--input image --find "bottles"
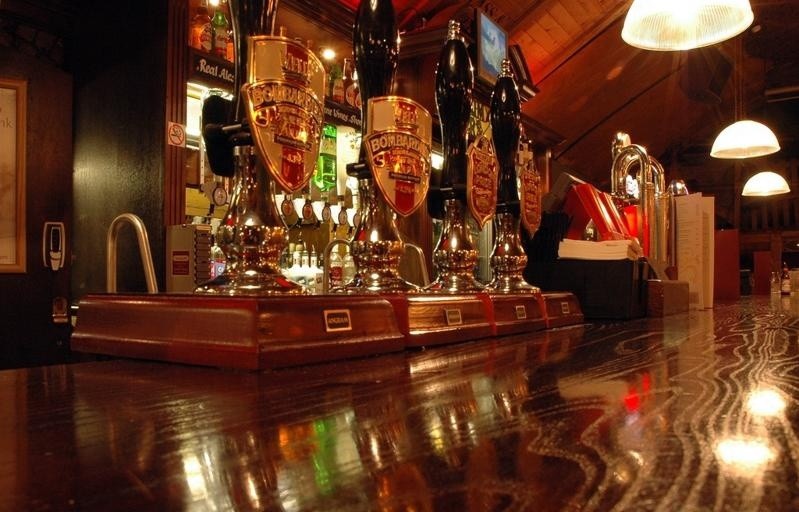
[331,59,345,103]
[330,241,343,287]
[351,66,361,118]
[780,267,791,295]
[210,3,228,59]
[226,30,235,63]
[344,247,357,286]
[279,26,331,99]
[342,57,355,108]
[189,6,213,53]
[769,271,782,295]
[283,238,323,271]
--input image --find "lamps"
[741,171,792,197]
[710,119,781,160]
[621,1,754,54]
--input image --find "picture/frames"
[0,79,29,272]
